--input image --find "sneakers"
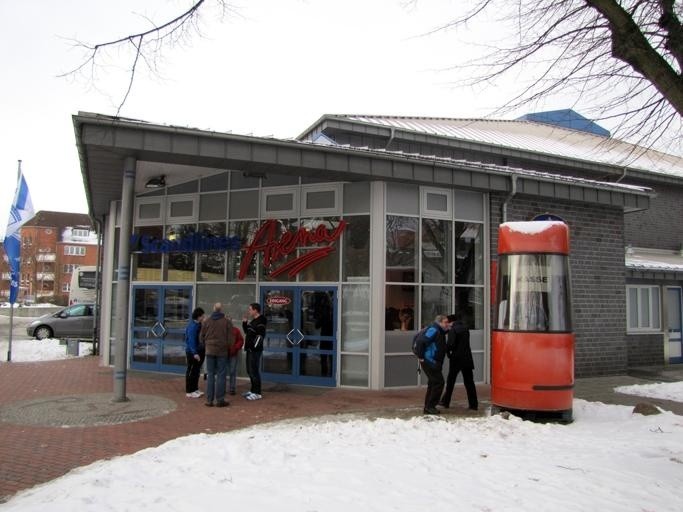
[204,399,229,407]
[438,400,450,409]
[241,390,263,401]
[186,390,205,398]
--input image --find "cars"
[170,252,258,276]
[132,295,320,375]
[24,295,37,305]
[25,301,99,343]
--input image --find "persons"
[438,314,479,411]
[285,291,332,377]
[412,314,449,414]
[199,302,234,407]
[185,307,206,398]
[240,303,267,400]
[225,313,244,395]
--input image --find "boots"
[424,404,441,415]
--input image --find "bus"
[64,265,105,312]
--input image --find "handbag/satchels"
[412,326,432,358]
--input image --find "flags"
[8,178,34,303]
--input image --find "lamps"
[144,172,165,190]
